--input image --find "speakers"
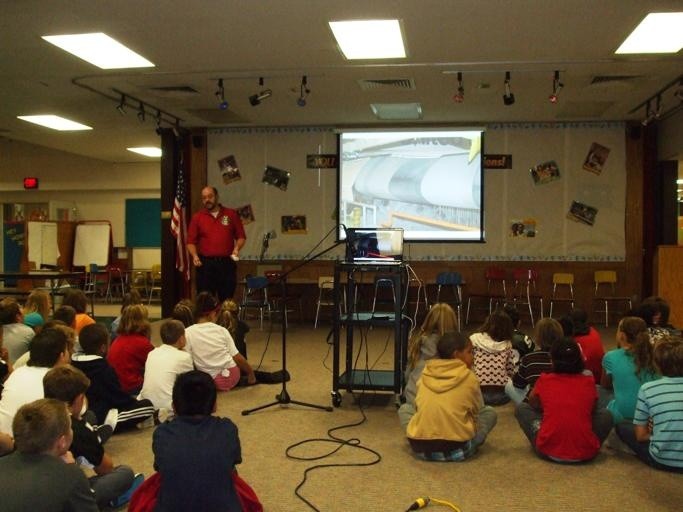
[631,126,641,139]
[193,136,202,148]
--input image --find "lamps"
[440,68,566,105]
[207,69,325,109]
[627,75,683,126]
[113,86,186,137]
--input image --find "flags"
[170,145,191,300]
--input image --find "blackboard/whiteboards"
[125,197,161,249]
[133,247,161,272]
[72,223,111,267]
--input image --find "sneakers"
[75,456,98,479]
[109,473,144,508]
[271,369,290,382]
[136,408,168,429]
[92,424,112,445]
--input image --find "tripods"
[241,241,345,415]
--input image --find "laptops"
[340,227,404,266]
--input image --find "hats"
[23,312,44,327]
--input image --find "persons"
[185,186,246,302]
[509,224,528,237]
[397,297,682,473]
[1,287,291,512]
[531,163,557,182]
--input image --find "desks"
[0,272,84,315]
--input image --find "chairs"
[85,264,165,319]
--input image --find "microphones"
[343,225,358,256]
